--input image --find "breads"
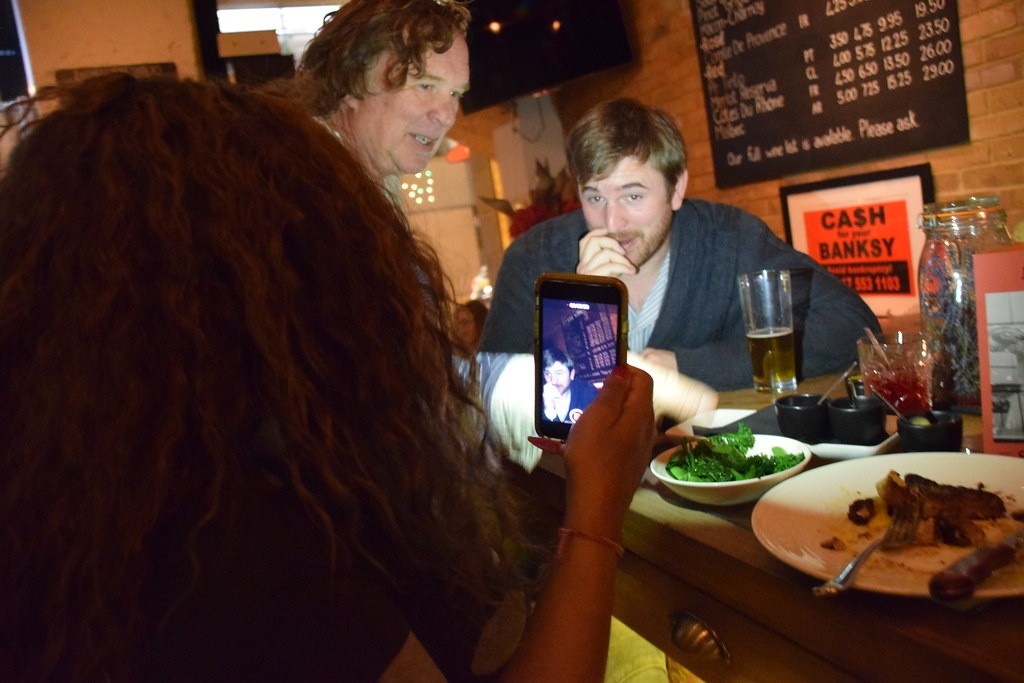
[882,471,1006,547]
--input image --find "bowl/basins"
[650,434,812,506]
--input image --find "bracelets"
[559,527,625,557]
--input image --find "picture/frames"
[780,161,938,317]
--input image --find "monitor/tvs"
[455,0,641,116]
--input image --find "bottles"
[918,194,1011,416]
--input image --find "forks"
[812,497,919,597]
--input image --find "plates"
[751,451,1024,599]
[664,408,899,463]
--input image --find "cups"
[896,411,963,453]
[775,393,832,444]
[827,395,886,445]
[735,268,798,394]
[857,333,932,416]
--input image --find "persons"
[297,0,716,469]
[0,70,662,683]
[540,347,600,424]
[452,300,488,348]
[483,97,887,392]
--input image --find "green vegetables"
[664,421,804,483]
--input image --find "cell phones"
[532,273,630,437]
